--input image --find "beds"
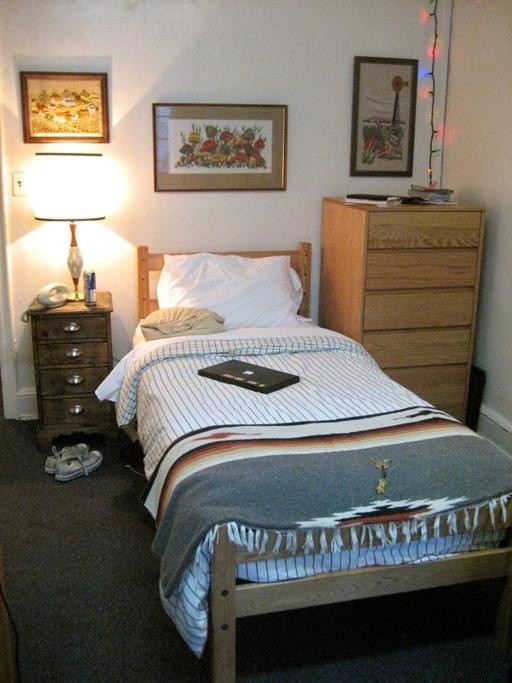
[127,240,509,681]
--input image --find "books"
[344,194,402,207]
[408,189,455,204]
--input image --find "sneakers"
[43,443,103,481]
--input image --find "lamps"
[29,151,110,300]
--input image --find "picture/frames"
[151,102,289,193]
[17,68,111,146]
[350,53,420,179]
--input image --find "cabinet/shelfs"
[320,194,487,428]
[26,289,117,455]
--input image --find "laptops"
[198,358,299,394]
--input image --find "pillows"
[154,250,303,333]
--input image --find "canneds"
[84,272,96,306]
